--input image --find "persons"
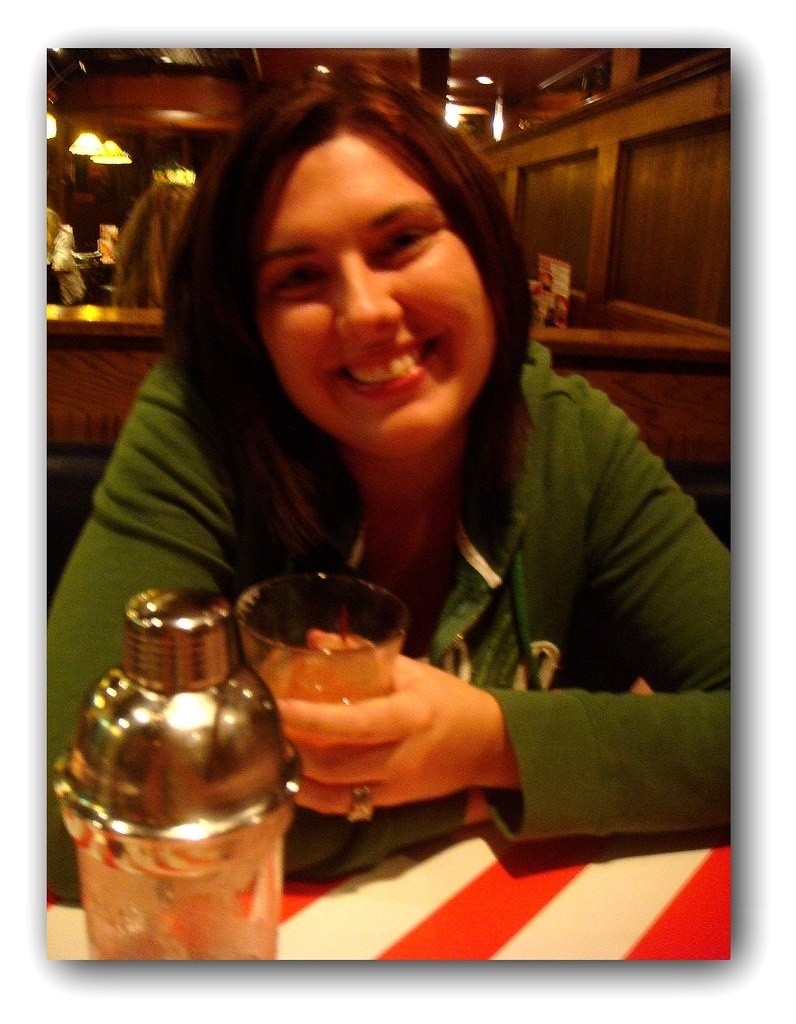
[46,63,730,904]
[112,180,194,307]
[47,206,87,307]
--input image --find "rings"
[347,785,373,822]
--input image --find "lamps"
[67,128,133,164]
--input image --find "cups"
[234,572,407,698]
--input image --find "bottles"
[54,587,301,960]
